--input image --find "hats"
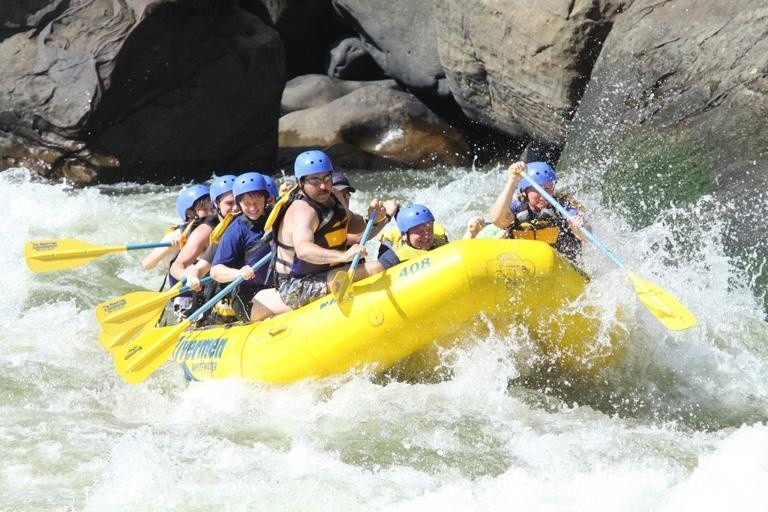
[332,172,355,193]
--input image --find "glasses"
[306,174,332,185]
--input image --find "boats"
[94,238,636,388]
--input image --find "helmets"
[516,162,555,191]
[396,204,435,232]
[176,172,278,220]
[294,149,333,178]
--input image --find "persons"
[490,159,593,269]
[144,171,397,325]
[376,205,487,271]
[272,151,387,312]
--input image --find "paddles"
[26,237,177,273]
[114,249,276,386]
[329,203,382,317]
[94,275,213,336]
[510,165,696,332]
[100,278,190,357]
